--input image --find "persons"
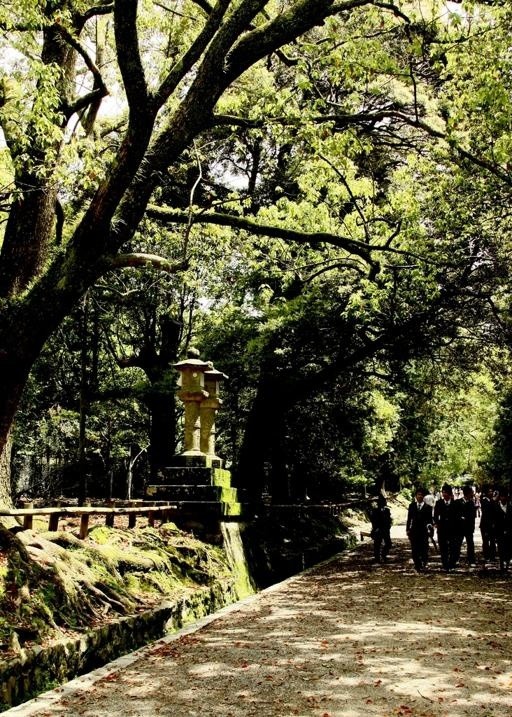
[406,474,512,571]
[369,494,394,563]
